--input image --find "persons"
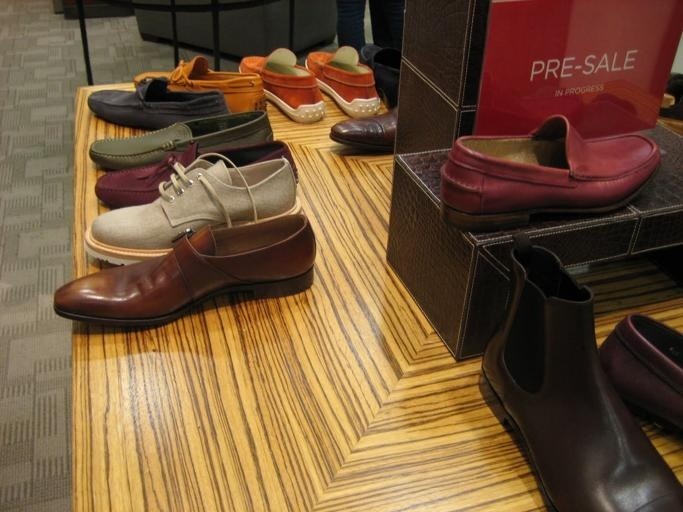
[336,0,404,51]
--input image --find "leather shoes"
[436,113,662,235]
[306,43,382,119]
[331,106,397,150]
[52,212,317,329]
[239,47,324,123]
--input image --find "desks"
[67,56,681,512]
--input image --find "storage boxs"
[378,120,682,370]
[391,1,498,170]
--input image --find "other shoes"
[359,43,402,102]
[81,42,303,267]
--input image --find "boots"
[475,231,683,512]
